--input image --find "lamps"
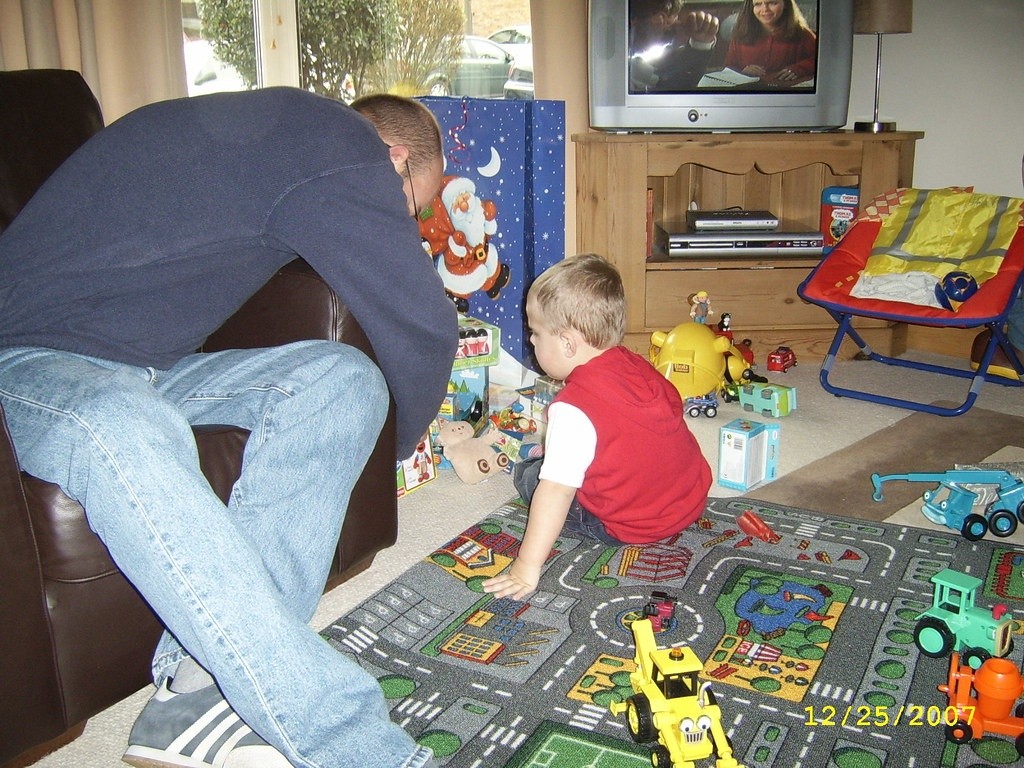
[852,0,913,130]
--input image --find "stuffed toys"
[437,421,509,485]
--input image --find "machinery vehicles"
[869,461,1024,543]
[912,567,1015,671]
[612,616,748,768]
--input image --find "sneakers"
[122,676,295,768]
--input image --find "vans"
[767,345,798,373]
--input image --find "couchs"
[0,69,398,767]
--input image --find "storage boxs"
[396,310,560,498]
[718,419,780,490]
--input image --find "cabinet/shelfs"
[568,129,932,361]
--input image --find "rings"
[789,70,792,72]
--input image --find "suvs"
[721,380,741,403]
[686,392,720,419]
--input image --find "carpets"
[318,490,1024,768]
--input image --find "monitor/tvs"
[588,0,853,133]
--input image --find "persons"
[723,0,816,81]
[690,291,713,324]
[629,0,720,90]
[481,252,713,603]
[0,87,460,768]
